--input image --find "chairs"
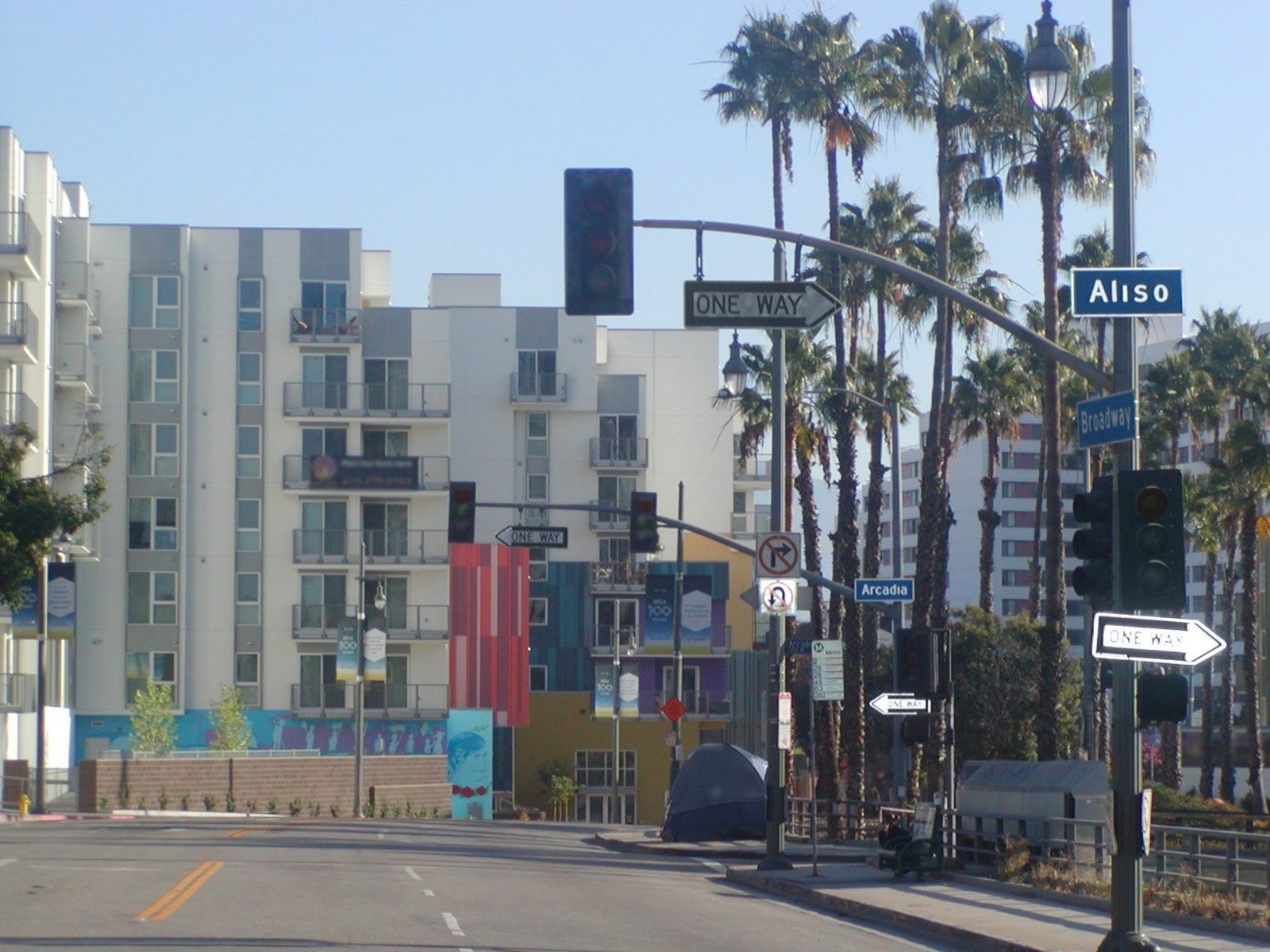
[340,315,357,334]
[292,314,309,333]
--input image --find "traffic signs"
[682,280,844,332]
[868,693,931,717]
[494,525,569,549]
[1090,609,1228,667]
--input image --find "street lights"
[609,593,639,826]
[722,242,793,869]
[1018,0,1161,952]
[350,541,387,819]
[804,386,908,813]
[31,475,76,815]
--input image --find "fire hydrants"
[16,794,32,818]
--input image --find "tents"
[659,741,769,846]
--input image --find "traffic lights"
[629,489,660,555]
[1117,468,1188,611]
[1071,474,1115,610]
[447,480,476,545]
[1135,672,1191,723]
[561,167,635,317]
